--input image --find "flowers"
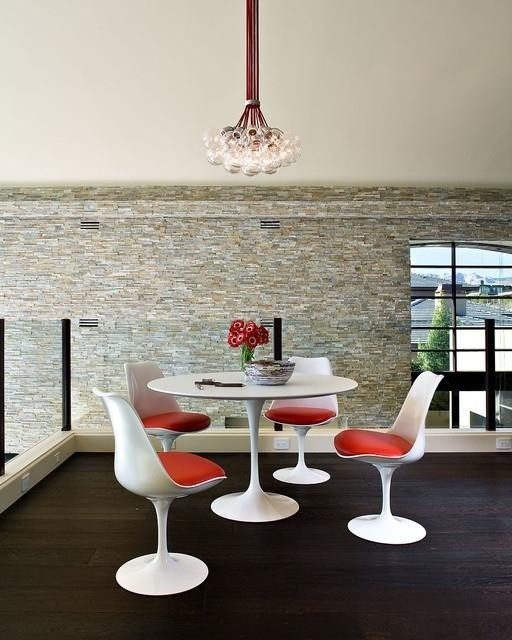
[226,317,270,369]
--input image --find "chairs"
[90,361,228,597]
[264,355,447,546]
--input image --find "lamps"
[200,0,306,182]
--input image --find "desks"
[147,371,359,524]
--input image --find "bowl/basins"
[243,360,296,386]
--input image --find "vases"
[239,344,254,372]
